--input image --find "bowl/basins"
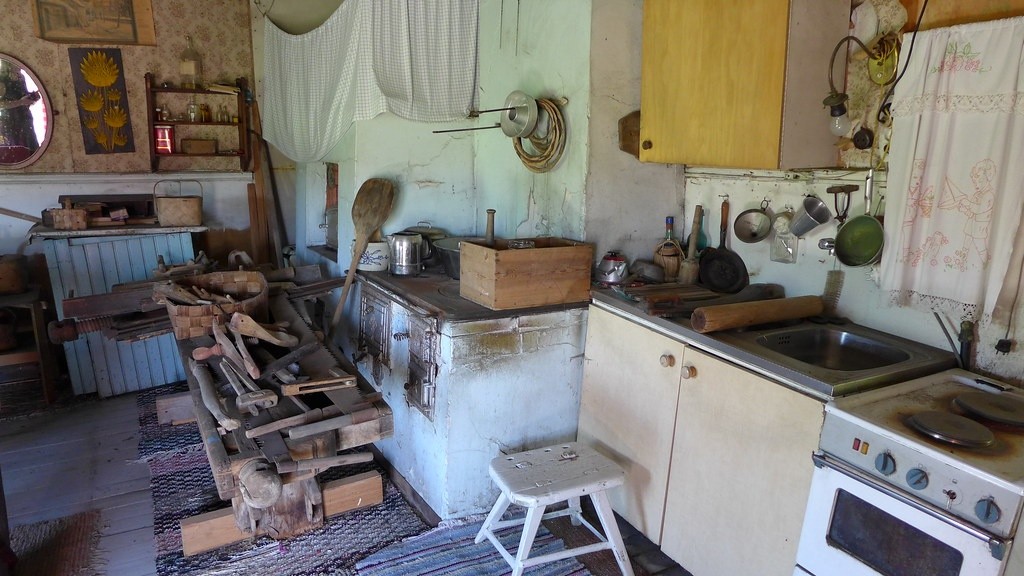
[351,239,390,271]
[403,221,448,267]
[432,236,502,280]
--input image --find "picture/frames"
[36,0,139,44]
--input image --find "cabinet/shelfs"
[145,71,251,172]
[638,0,852,170]
[0,296,59,418]
[575,304,832,576]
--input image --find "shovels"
[326,177,394,341]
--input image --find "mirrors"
[0,52,53,170]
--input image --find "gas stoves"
[818,367,1024,539]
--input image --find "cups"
[787,197,830,237]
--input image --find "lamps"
[823,34,898,136]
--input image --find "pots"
[834,168,885,267]
[699,200,750,294]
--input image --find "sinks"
[752,323,913,371]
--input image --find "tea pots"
[594,251,629,285]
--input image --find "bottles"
[661,216,675,246]
[179,35,203,93]
[161,95,238,123]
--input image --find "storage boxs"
[460,237,593,310]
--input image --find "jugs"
[385,232,422,277]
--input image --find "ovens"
[794,450,1012,576]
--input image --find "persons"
[0,60,40,151]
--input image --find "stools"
[475,442,635,576]
